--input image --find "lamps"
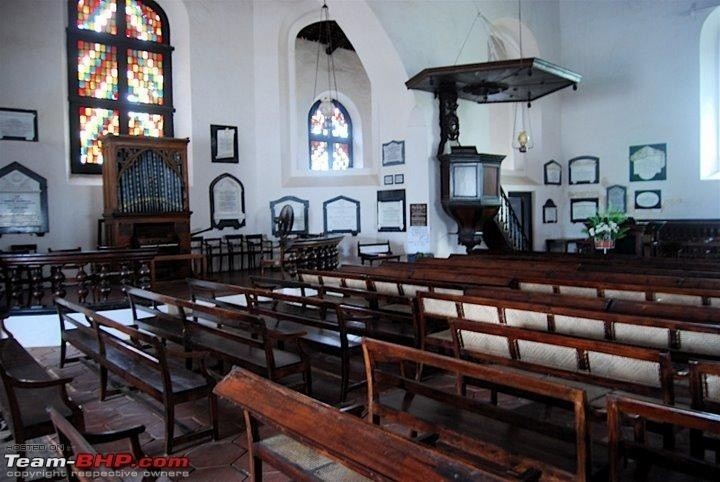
[314,1,346,124]
[509,1,537,154]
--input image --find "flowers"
[579,201,633,242]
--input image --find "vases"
[592,234,617,254]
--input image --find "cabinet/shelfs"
[435,148,508,212]
